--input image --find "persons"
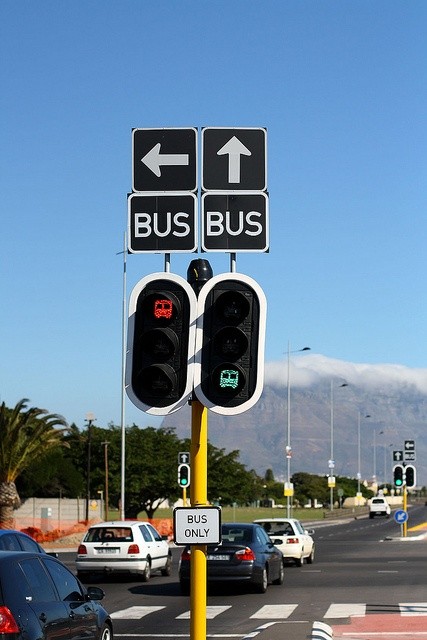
[263,523,272,533]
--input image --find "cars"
[0,531,58,559]
[75,521,172,581]
[0,552,113,640]
[368,498,390,518]
[178,523,284,591]
[253,518,315,566]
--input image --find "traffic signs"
[127,127,198,253]
[404,440,415,462]
[178,452,189,464]
[172,507,220,545]
[395,510,408,524]
[392,451,402,461]
[200,126,269,253]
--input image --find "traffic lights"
[124,272,196,415]
[392,465,404,488]
[404,465,416,488]
[177,464,190,488]
[193,273,266,415]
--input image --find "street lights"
[329,379,347,510]
[357,411,370,505]
[384,443,393,495]
[83,413,97,520]
[283,339,310,519]
[373,429,385,497]
[101,441,112,521]
